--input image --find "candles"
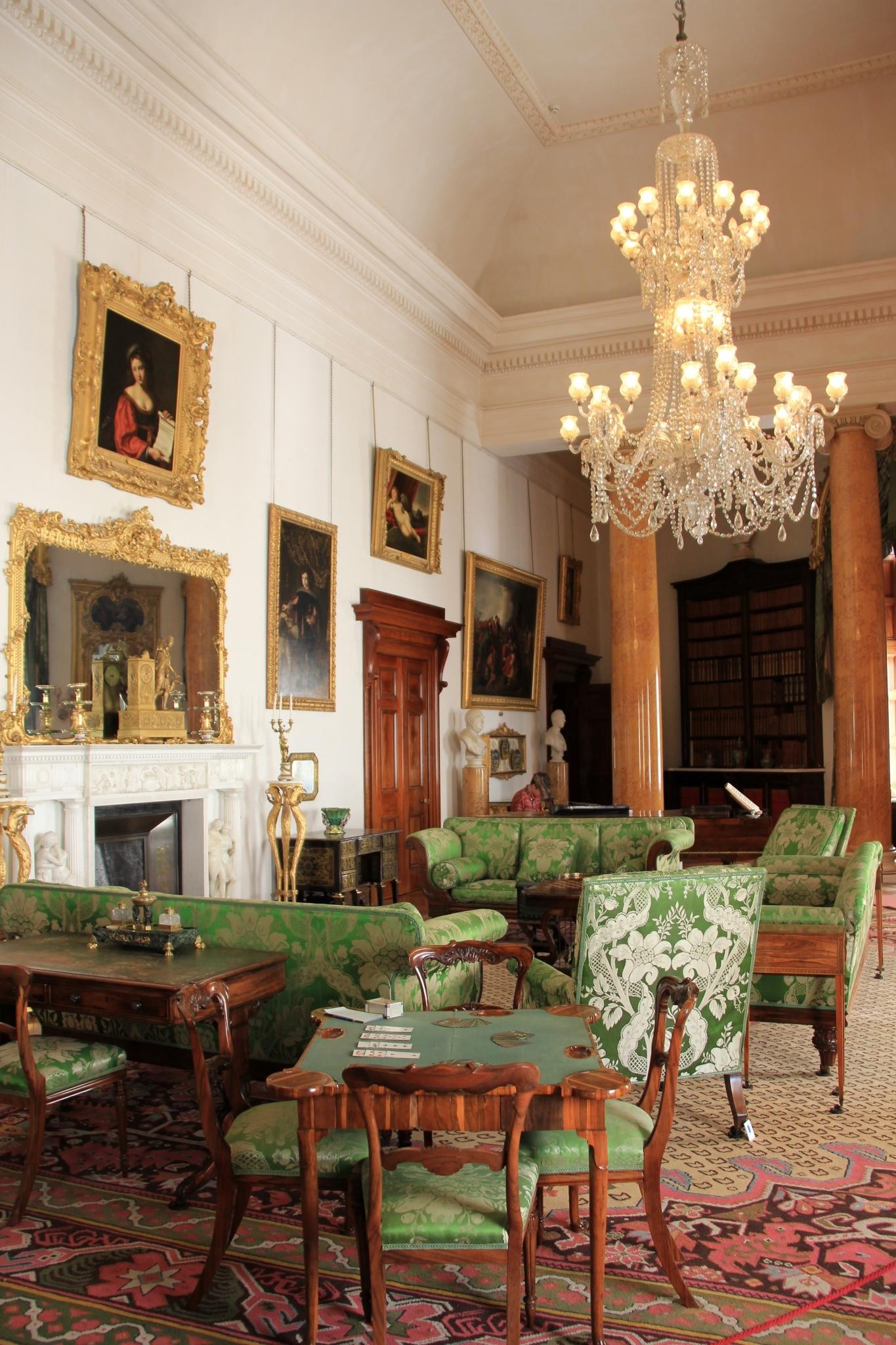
[273,692,293,720]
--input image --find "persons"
[459,709,488,767]
[510,772,552,812]
[151,636,181,710]
[543,709,569,762]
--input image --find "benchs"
[406,815,695,919]
[742,841,885,1114]
[0,879,508,1080]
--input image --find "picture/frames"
[266,504,338,712]
[461,551,548,711]
[370,447,447,575]
[557,555,582,626]
[66,260,216,508]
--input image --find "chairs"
[173,865,768,1345]
[762,804,857,857]
[0,964,128,1224]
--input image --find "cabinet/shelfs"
[670,556,823,769]
[275,828,402,906]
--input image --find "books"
[685,585,810,768]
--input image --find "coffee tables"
[515,872,596,967]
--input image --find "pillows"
[616,857,645,873]
[432,857,488,889]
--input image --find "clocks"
[117,651,187,744]
[84,651,124,739]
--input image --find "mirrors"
[0,506,235,745]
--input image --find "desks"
[0,930,289,1210]
[665,768,824,816]
[266,1004,633,1345]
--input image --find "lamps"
[560,0,848,550]
